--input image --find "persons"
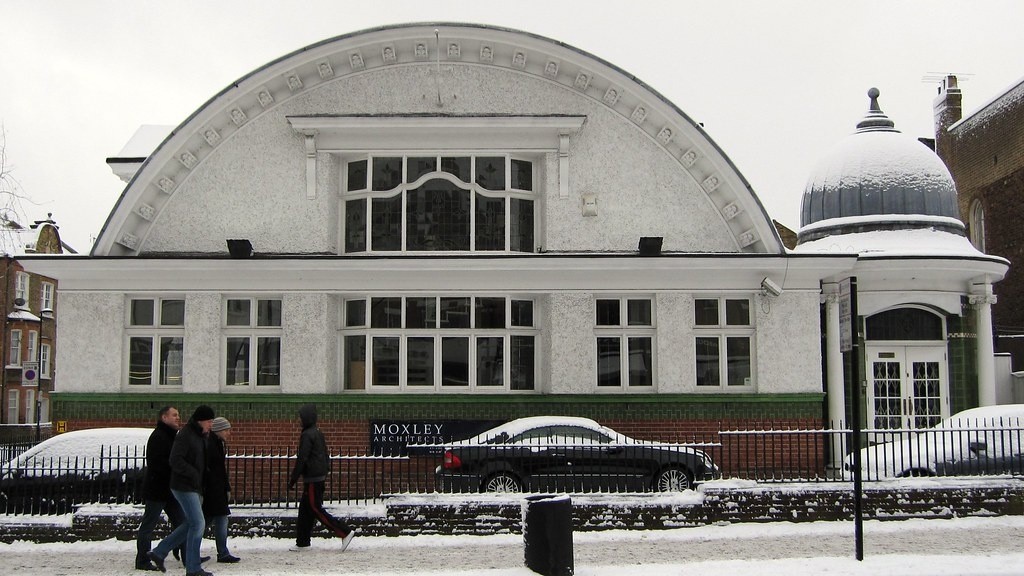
[135,405,211,571]
[173,417,241,564]
[287,406,355,553]
[147,406,215,576]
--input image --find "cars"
[435,416,720,495]
[843,403,1024,480]
[0,428,181,514]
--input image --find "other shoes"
[200,556,210,563]
[341,530,354,552]
[217,555,241,563]
[135,562,158,571]
[186,568,213,576]
[289,544,312,552]
[148,550,167,574]
[172,547,181,561]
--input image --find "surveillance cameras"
[760,276,783,296]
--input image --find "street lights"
[37,308,54,442]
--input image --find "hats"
[211,417,231,432]
[194,405,215,422]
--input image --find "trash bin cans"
[521,493,574,576]
